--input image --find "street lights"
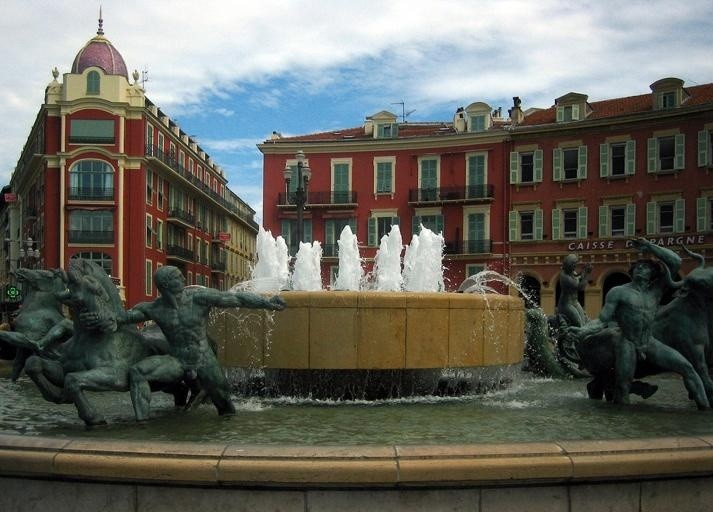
[19,237,40,295]
[283,150,312,250]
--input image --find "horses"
[24,256,218,426]
[0,267,75,385]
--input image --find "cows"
[557,242,713,400]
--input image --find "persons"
[555,254,595,329]
[565,237,710,412]
[125,266,286,422]
